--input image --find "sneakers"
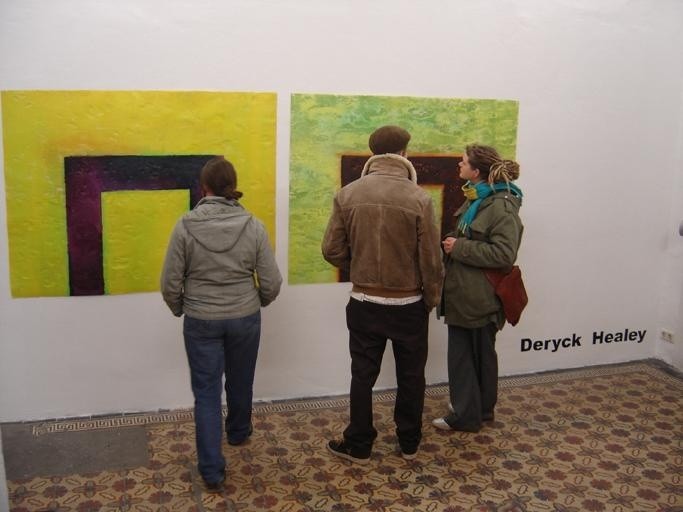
[396,429,420,460]
[327,439,371,466]
[206,467,227,494]
[432,403,455,430]
[228,421,253,445]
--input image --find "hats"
[368,125,411,154]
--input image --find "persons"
[318,125,449,466]
[159,154,282,489]
[432,140,524,433]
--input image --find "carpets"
[2,412,150,479]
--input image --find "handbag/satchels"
[483,265,529,327]
